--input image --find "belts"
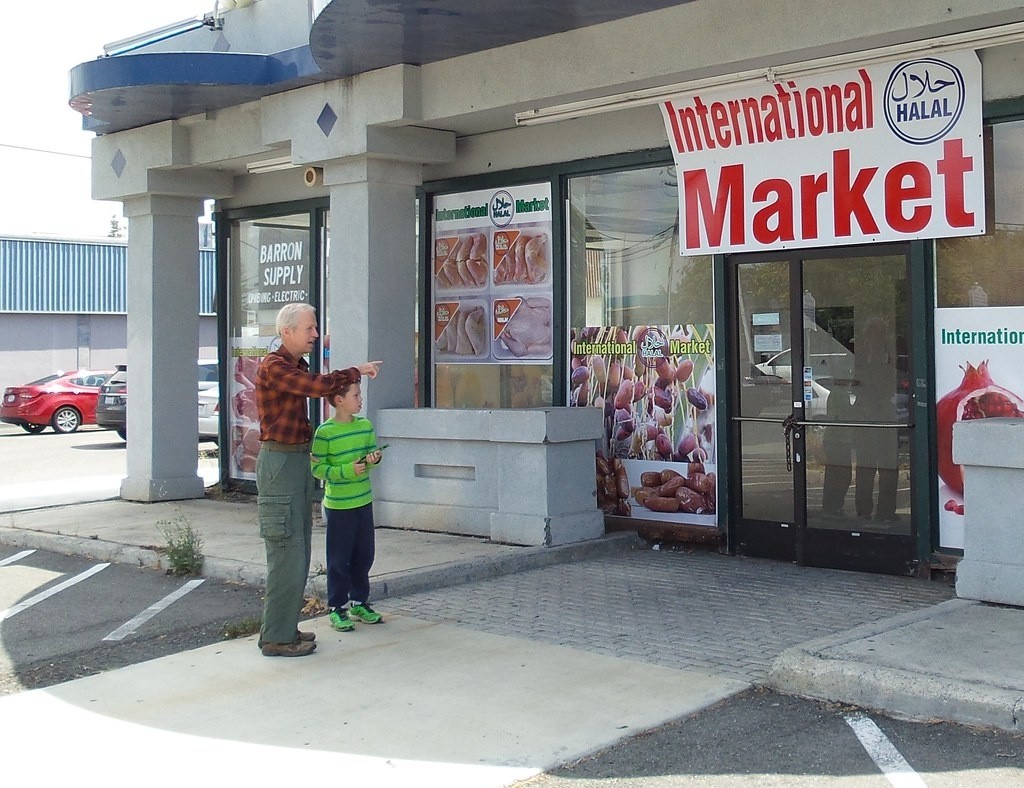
[260,442,311,454]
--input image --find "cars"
[95,364,127,440]
[0,368,113,435]
[752,365,831,441]
[197,358,219,447]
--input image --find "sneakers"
[876,513,900,522]
[329,607,355,632]
[855,513,871,521]
[823,508,847,520]
[349,602,382,624]
[262,641,316,657]
[258,630,315,648]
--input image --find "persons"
[254,303,384,657]
[821,318,901,522]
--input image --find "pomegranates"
[935,358,1024,515]
